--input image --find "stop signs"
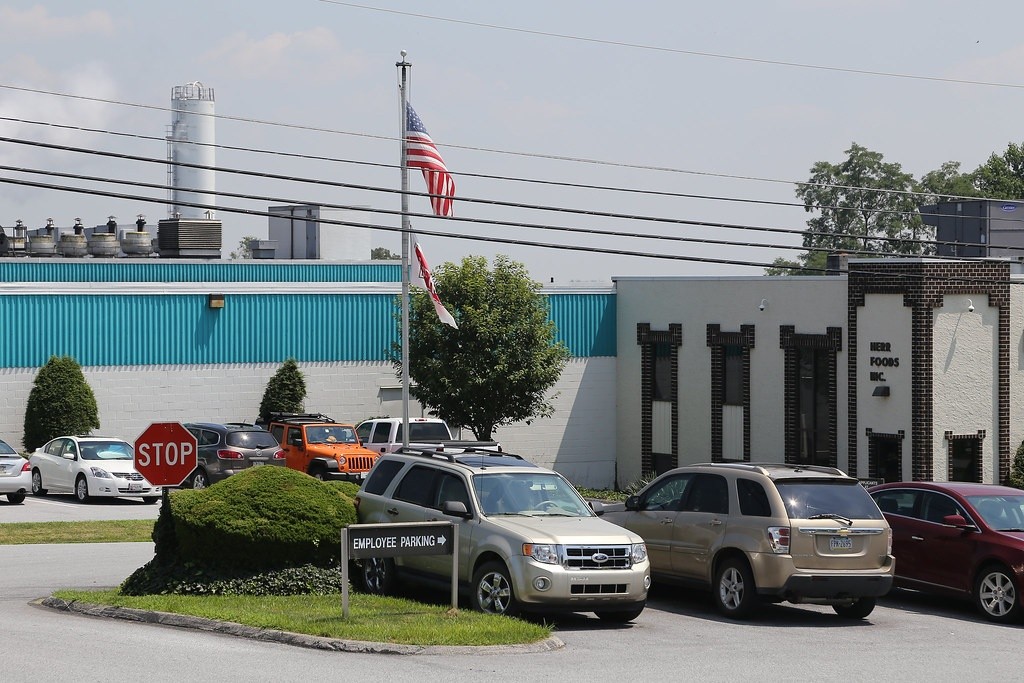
[133,421,198,486]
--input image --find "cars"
[0,437,31,505]
[866,480,1024,630]
[29,435,163,504]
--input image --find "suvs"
[352,441,652,629]
[180,422,287,490]
[266,411,381,481]
[596,459,896,620]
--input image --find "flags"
[410,226,459,329]
[406,100,456,217]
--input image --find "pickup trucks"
[347,417,502,460]
